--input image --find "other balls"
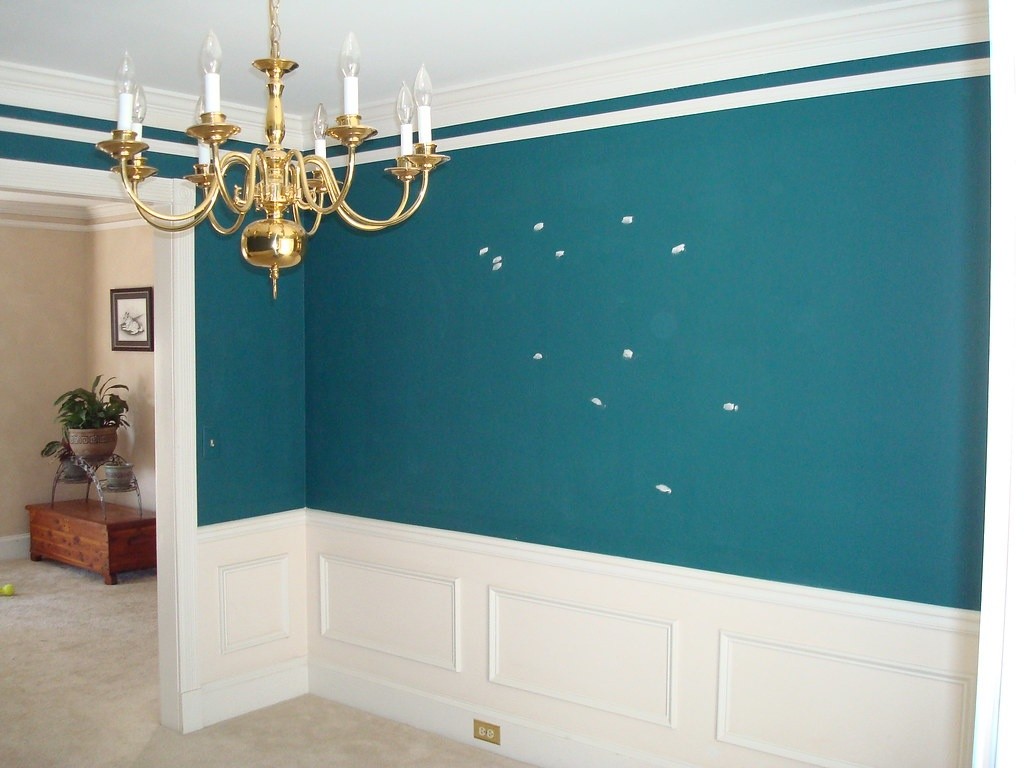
[4,584,14,595]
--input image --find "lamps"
[96,0,451,299]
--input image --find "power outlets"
[474,719,500,745]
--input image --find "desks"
[25,499,156,585]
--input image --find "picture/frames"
[109,287,154,352]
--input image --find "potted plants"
[104,456,135,490]
[54,374,130,461]
[41,440,89,481]
